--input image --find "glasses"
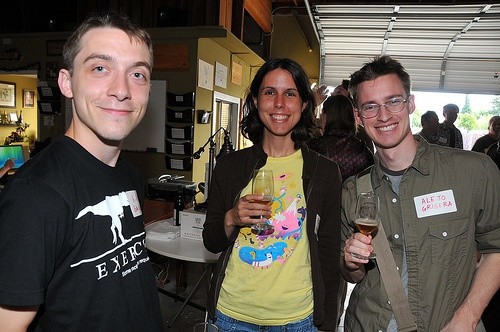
[358,96,409,119]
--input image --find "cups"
[193,323,219,332]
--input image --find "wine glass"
[251,170,274,230]
[351,193,380,259]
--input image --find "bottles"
[173,185,185,226]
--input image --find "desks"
[143,217,222,332]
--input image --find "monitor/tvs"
[0,145,25,170]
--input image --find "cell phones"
[342,79,349,91]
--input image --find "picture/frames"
[0,80,56,134]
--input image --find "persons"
[302,76,375,163]
[471,116,500,165]
[413,110,440,145]
[306,96,376,330]
[202,57,344,332]
[1,11,163,332]
[437,105,462,148]
[338,57,500,332]
